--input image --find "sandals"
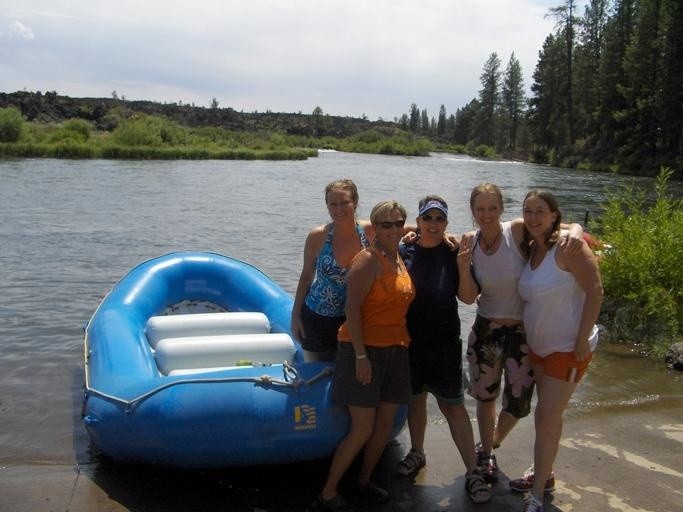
[394,450,427,477]
[479,450,497,482]
[318,495,357,511]
[358,482,390,504]
[474,438,500,453]
[465,469,490,502]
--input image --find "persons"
[511,190,604,512]
[388,196,478,479]
[293,178,423,360]
[463,184,583,507]
[314,202,420,511]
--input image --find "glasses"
[378,220,405,227]
[421,215,446,222]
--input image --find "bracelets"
[355,352,367,359]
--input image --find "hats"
[418,200,449,215]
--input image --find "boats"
[85,250,410,469]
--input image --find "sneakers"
[509,472,554,492]
[522,498,543,512]
